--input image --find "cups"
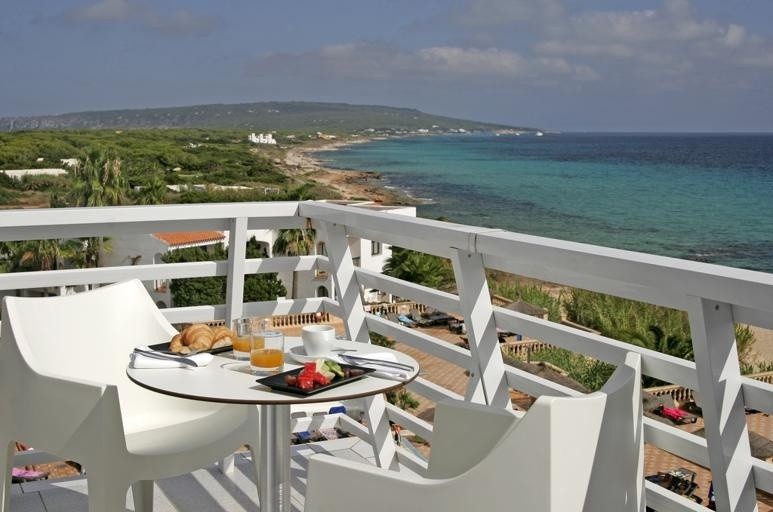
[233,317,267,361]
[302,325,335,356]
[250,331,285,377]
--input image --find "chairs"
[305,351,647,512]
[0,279,262,512]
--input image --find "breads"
[168,322,234,355]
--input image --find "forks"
[337,352,414,371]
[133,348,198,370]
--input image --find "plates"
[288,345,347,364]
[255,364,376,395]
[148,340,233,358]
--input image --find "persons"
[10,444,41,479]
[656,473,672,488]
[657,402,689,422]
